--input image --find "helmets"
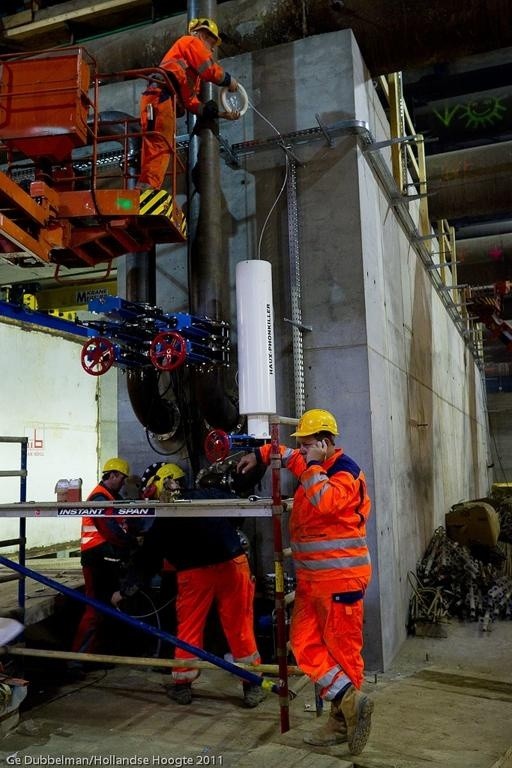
[103,458,130,476]
[147,472,173,498]
[156,464,186,479]
[289,409,338,437]
[188,18,222,47]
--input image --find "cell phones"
[322,439,329,448]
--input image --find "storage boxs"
[445,502,500,548]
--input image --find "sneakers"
[302,699,347,747]
[175,684,191,704]
[339,684,374,753]
[244,682,266,706]
[134,182,156,190]
[67,659,87,680]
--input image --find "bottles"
[55,477,83,502]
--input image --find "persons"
[139,461,279,704]
[138,15,242,193]
[67,454,131,670]
[235,404,376,757]
